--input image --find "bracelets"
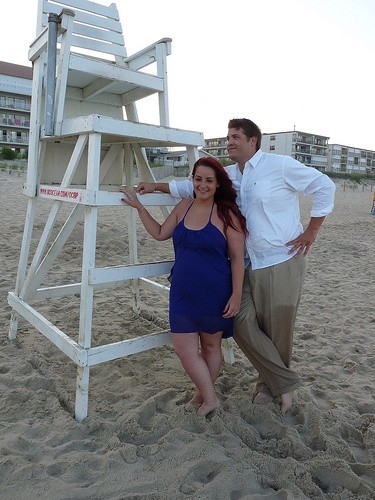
[138,206,144,213]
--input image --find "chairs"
[27,0,173,139]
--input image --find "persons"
[117,156,250,418]
[135,118,336,416]
